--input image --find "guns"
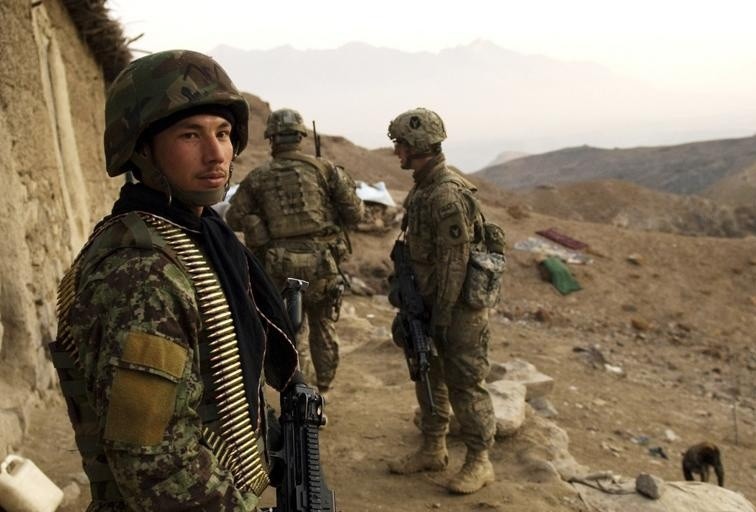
[311,122,323,158]
[270,276,336,508]
[391,239,439,419]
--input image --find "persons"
[45,49,296,511]
[386,106,496,493]
[223,110,359,428]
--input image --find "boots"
[448,449,495,493]
[388,434,449,476]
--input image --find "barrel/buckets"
[0,454,63,511]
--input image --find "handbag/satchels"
[467,251,505,310]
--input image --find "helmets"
[387,107,446,156]
[103,49,249,177]
[264,108,307,142]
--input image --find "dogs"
[679,440,724,487]
